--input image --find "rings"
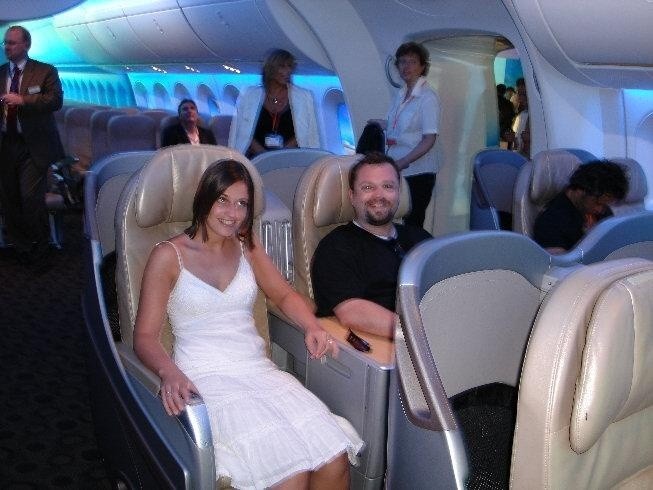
[164,391,171,395]
[326,339,332,344]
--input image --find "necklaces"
[266,87,285,104]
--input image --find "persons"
[533,159,629,255]
[366,42,440,229]
[224,48,320,162]
[496,77,530,160]
[308,151,439,340]
[160,98,219,148]
[132,157,368,490]
[0,25,66,267]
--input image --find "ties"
[5,67,20,147]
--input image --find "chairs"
[56,103,653,489]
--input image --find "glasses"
[1,39,24,47]
